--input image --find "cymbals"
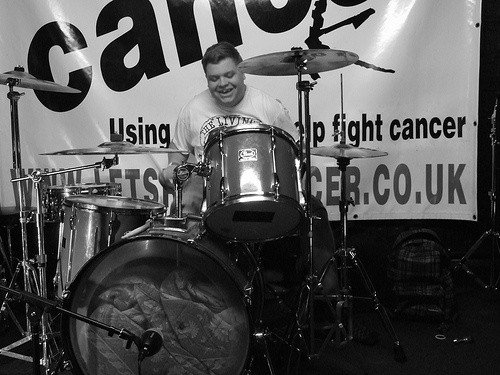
[310,143,388,159]
[1,71,83,94]
[38,139,189,156]
[236,50,360,76]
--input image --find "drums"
[55,225,266,375]
[54,194,166,302]
[201,121,305,246]
[46,184,122,222]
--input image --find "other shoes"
[341,318,378,345]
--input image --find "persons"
[167,41,379,345]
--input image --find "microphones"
[138,328,164,361]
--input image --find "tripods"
[0,78,120,375]
[453,100,500,295]
[279,56,406,375]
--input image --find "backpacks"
[386,219,456,323]
